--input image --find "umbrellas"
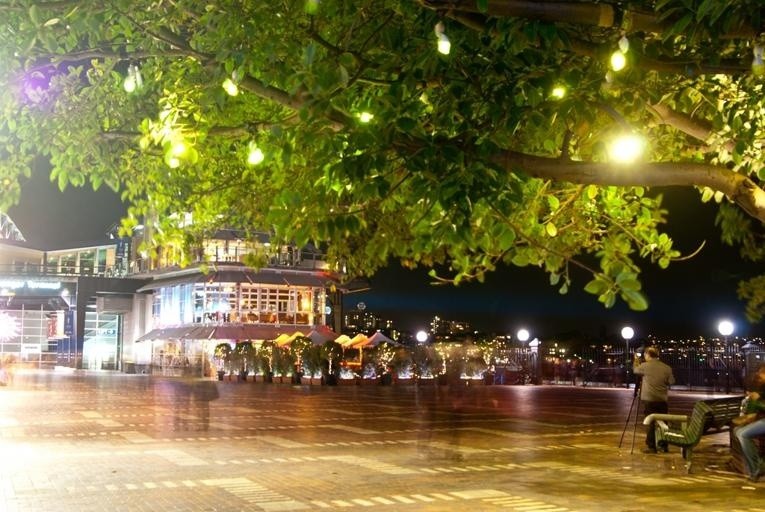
[270,327,399,364]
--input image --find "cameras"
[636,352,644,357]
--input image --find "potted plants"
[214,336,507,386]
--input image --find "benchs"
[655,396,760,475]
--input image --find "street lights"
[621,326,634,388]
[718,321,734,394]
[517,329,530,384]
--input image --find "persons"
[733,362,765,483]
[633,346,676,454]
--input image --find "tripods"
[618,361,643,455]
